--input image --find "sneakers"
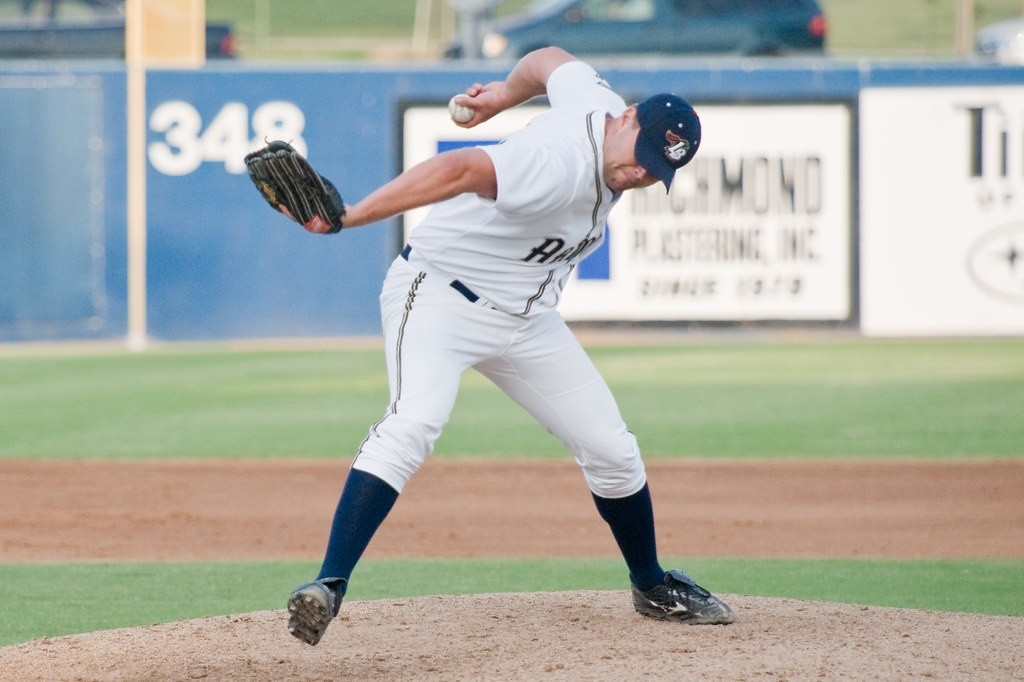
[632,567,736,624]
[287,577,347,646]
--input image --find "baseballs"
[448,93,474,122]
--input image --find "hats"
[634,94,701,194]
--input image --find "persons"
[244,46,737,647]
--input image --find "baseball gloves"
[244,140,348,234]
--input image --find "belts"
[400,244,480,303]
